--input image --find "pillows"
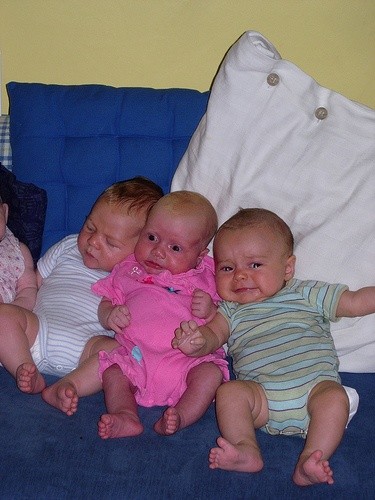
[7,81,212,260]
[170,31,375,373]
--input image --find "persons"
[171,208,375,485]
[0,190,37,312]
[92,190,229,439]
[0,176,165,415]
